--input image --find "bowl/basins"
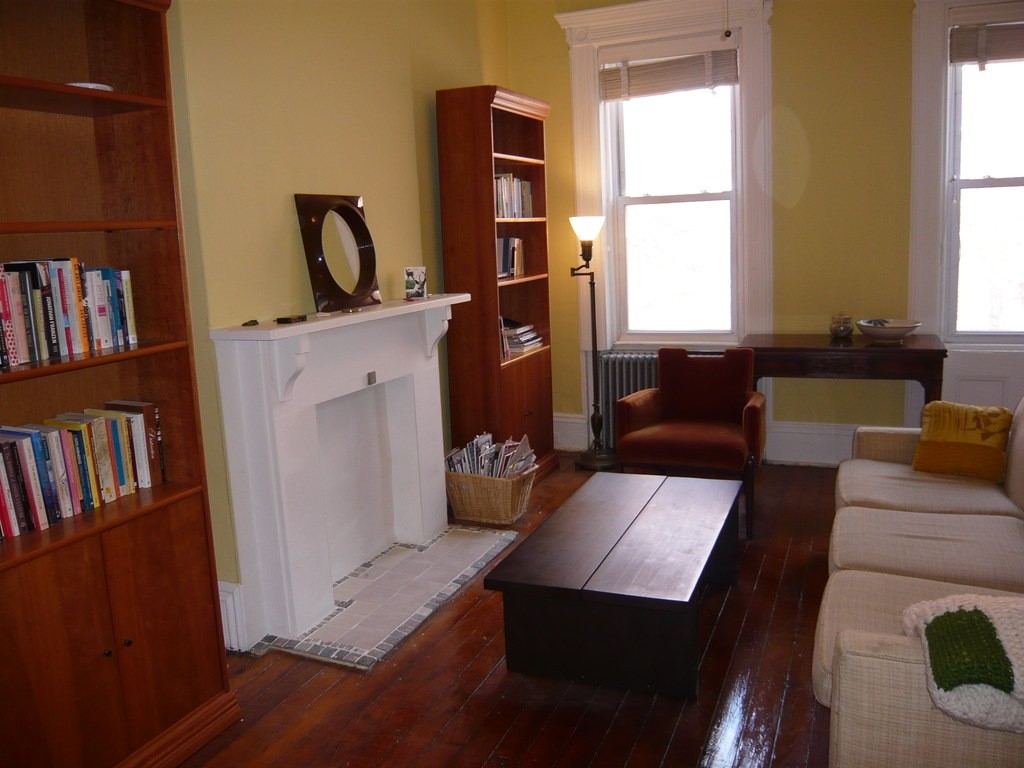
[856,319,922,342]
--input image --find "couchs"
[811,394,1024,768]
[615,347,766,540]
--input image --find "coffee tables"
[483,471,743,704]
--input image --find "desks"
[738,333,948,426]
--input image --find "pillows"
[900,592,1024,734]
[912,400,1013,482]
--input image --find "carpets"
[246,524,517,670]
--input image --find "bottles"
[830,315,854,338]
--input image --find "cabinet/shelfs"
[436,84,561,487]
[0,0,228,768]
[208,290,472,638]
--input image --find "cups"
[404,266,427,301]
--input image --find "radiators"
[598,351,659,450]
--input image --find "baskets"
[446,463,539,525]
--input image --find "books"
[443,433,537,478]
[498,238,524,278]
[0,399,167,540]
[0,256,138,368]
[494,173,532,218]
[504,324,544,352]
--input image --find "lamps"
[569,215,616,471]
